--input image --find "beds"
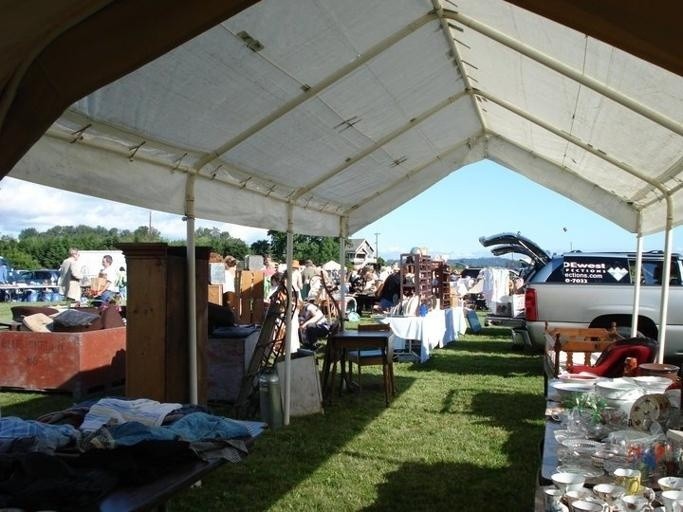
[543,320,629,398]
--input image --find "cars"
[479,230,683,358]
[461,266,519,279]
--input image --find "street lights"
[560,224,573,251]
[512,230,522,260]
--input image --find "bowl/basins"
[550,363,680,406]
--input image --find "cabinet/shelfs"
[430,261,451,310]
[400,253,433,312]
[112,241,212,408]
[1,324,127,391]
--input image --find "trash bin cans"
[42,292,59,301]
[26,290,37,302]
[15,292,23,301]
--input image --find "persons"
[115,266,127,300]
[207,247,464,352]
[58,247,83,308]
[510,267,529,295]
[88,255,119,310]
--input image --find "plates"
[553,388,683,479]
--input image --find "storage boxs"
[510,293,526,318]
[491,299,510,316]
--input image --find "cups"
[546,467,683,512]
[420,303,427,317]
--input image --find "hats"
[292,260,302,269]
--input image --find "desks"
[442,306,467,345]
[545,377,571,422]
[538,422,667,491]
[536,482,592,511]
[328,330,396,407]
[369,309,446,363]
[0,283,61,302]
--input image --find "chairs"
[566,337,658,377]
[348,322,396,396]
